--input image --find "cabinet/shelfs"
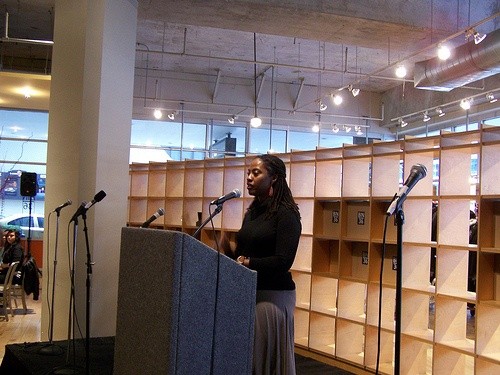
[127,124,500,375]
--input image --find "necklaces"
[1,246,12,257]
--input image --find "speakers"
[20,172,38,196]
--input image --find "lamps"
[228,115,238,124]
[354,125,364,137]
[423,110,431,121]
[438,108,445,117]
[343,124,351,133]
[330,93,342,105]
[489,92,498,104]
[332,124,339,133]
[167,110,179,121]
[400,118,408,127]
[317,100,328,113]
[469,28,488,45]
[347,85,361,98]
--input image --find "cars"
[0,212,44,240]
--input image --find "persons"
[220,154,302,375]
[0,229,24,308]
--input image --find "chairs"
[11,257,40,312]
[0,261,20,322]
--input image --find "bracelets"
[8,263,10,267]
[237,256,243,264]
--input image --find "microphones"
[78,191,106,215]
[70,200,86,221]
[54,200,72,211]
[386,164,428,215]
[210,189,242,204]
[141,207,166,228]
